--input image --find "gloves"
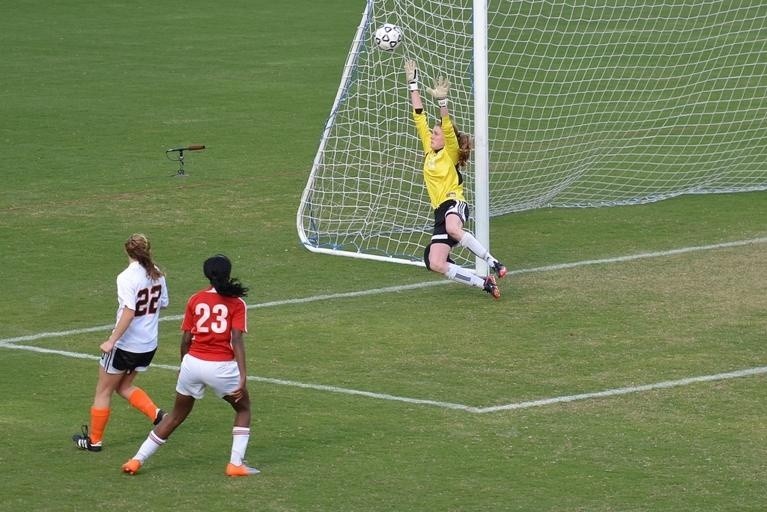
[426,75,450,107]
[403,60,419,91]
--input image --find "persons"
[403,58,507,300]
[120,253,260,476]
[71,232,170,451]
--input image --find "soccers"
[373,23,403,50]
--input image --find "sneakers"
[491,261,509,280]
[481,274,500,299]
[224,462,261,476]
[153,409,168,427]
[72,434,102,452]
[120,459,141,475]
